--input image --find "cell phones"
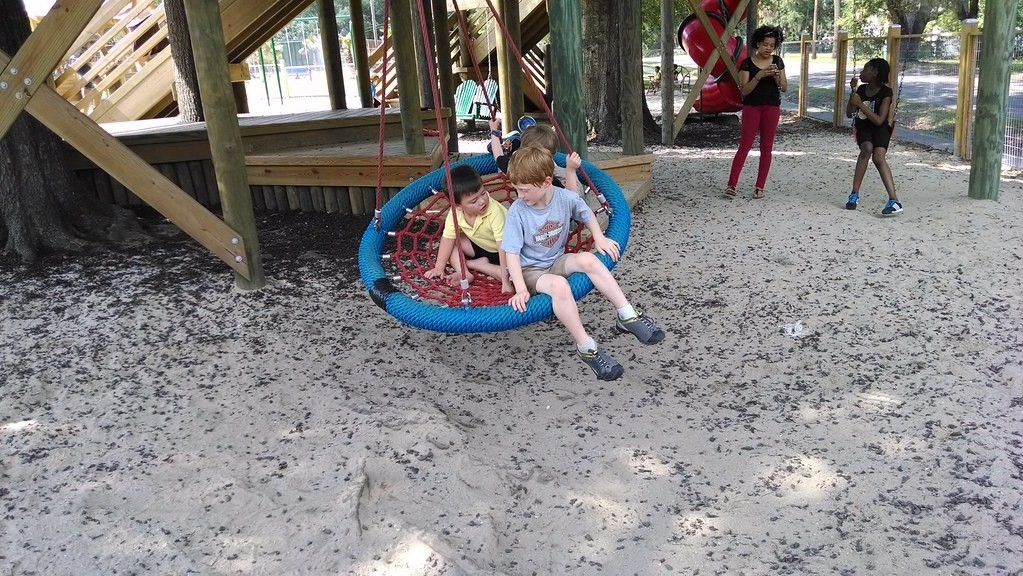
[770,64,778,72]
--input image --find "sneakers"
[882,199,903,216]
[615,311,665,345]
[846,193,859,210]
[576,340,624,382]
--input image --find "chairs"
[455,79,500,131]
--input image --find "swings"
[850,0,916,149]
[355,1,632,336]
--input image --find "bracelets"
[754,75,760,81]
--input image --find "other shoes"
[725,186,736,198]
[753,186,765,199]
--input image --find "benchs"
[649,65,691,94]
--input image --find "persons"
[487,116,582,190]
[370,75,381,109]
[422,165,508,288]
[723,24,787,199]
[846,57,903,215]
[499,145,666,382]
[76,0,169,115]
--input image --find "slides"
[677,0,758,116]
